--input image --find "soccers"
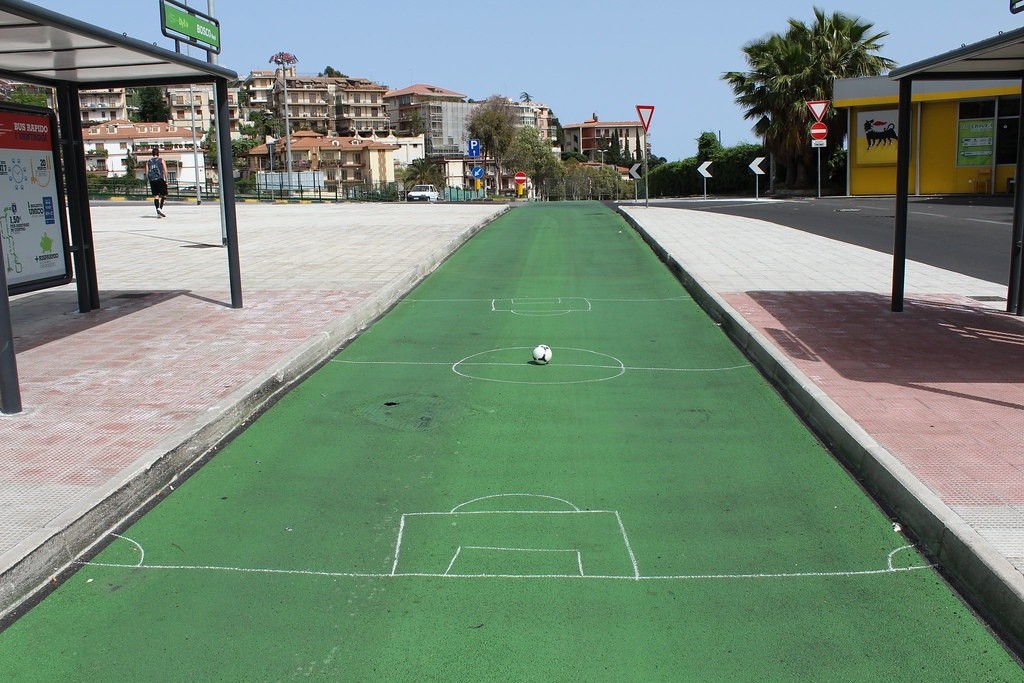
[532,344,552,365]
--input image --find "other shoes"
[156,214,161,218]
[157,207,166,217]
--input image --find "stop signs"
[515,172,527,185]
[810,123,827,140]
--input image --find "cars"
[407,184,440,203]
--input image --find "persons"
[146,147,168,218]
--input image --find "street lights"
[269,52,300,195]
